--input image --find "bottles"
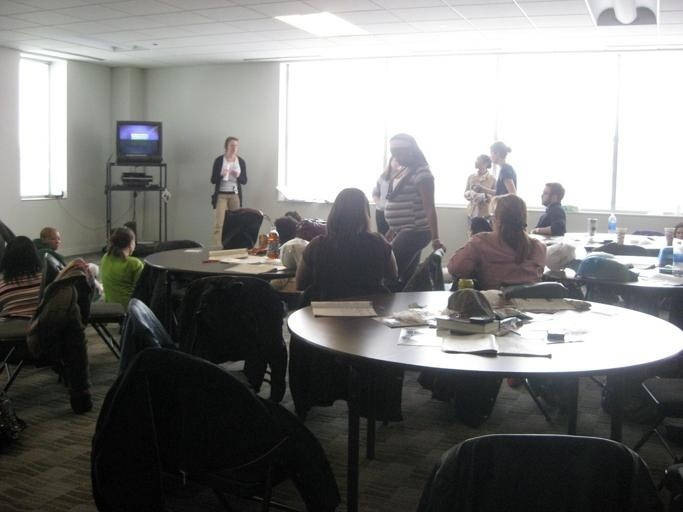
[608,212,616,234]
[223,164,230,183]
[658,228,683,275]
[259,227,280,258]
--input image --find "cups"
[589,218,597,237]
[616,227,627,246]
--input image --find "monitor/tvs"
[115,121,162,163]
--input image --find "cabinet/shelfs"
[106,162,168,246]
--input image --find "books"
[507,297,578,312]
[437,332,554,358]
[434,307,521,335]
[207,247,288,274]
[308,300,379,317]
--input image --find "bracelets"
[432,238,440,243]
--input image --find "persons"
[371,155,402,236]
[294,187,401,310]
[384,132,446,278]
[462,154,498,234]
[0,235,44,321]
[97,225,144,311]
[447,194,546,290]
[209,136,247,244]
[527,181,566,236]
[489,141,518,194]
[673,223,683,240]
[33,226,103,302]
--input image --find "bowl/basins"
[546,261,572,272]
